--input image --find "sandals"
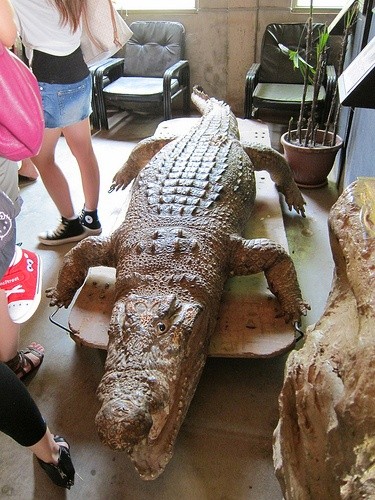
[4,342,45,387]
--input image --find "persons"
[0,0,75,381]
[6,0,133,245]
[0,362,75,490]
[16,37,38,187]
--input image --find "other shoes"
[18,174,37,186]
[36,433,75,490]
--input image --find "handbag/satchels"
[0,42,45,162]
[80,0,133,68]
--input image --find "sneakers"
[1,249,43,325]
[37,216,87,246]
[56,203,102,235]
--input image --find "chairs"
[95,20,190,130]
[245,22,336,129]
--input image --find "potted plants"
[267,0,365,187]
